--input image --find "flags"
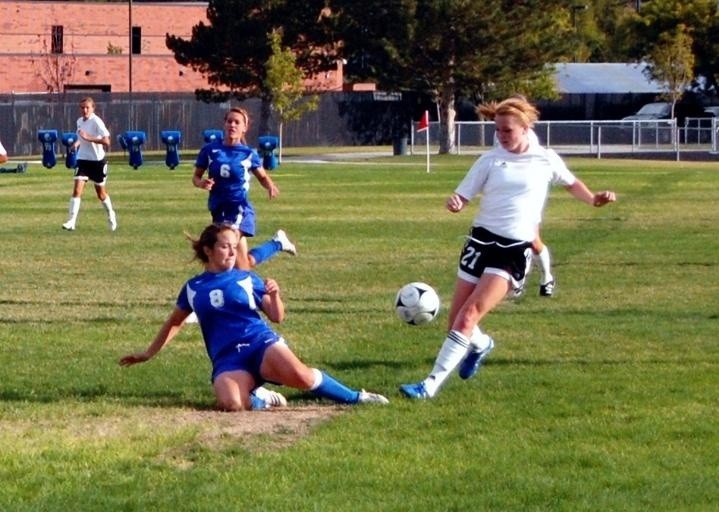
[415,113,429,133]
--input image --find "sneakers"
[400,384,429,399]
[273,228,296,254]
[357,389,390,405]
[252,385,287,407]
[458,335,493,379]
[61,219,76,230]
[539,275,555,296]
[107,211,116,231]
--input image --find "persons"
[191,105,299,271]
[0,140,9,168]
[60,97,117,232]
[396,97,617,401]
[492,91,556,298]
[114,224,392,411]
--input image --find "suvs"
[617,102,705,143]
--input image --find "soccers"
[393,281,440,325]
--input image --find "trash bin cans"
[391,134,408,155]
